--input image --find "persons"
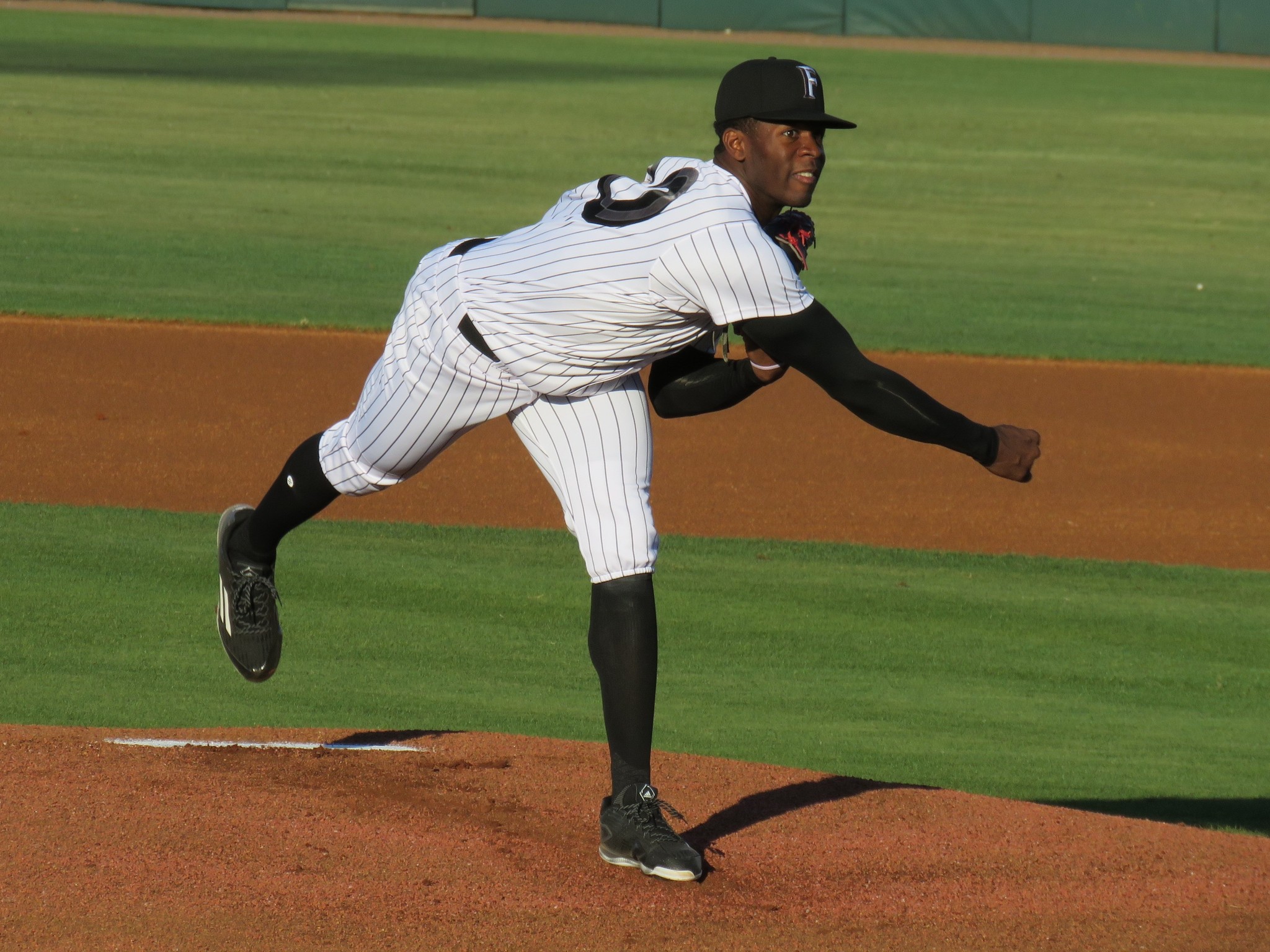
[217,57,1047,883]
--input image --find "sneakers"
[216,502,283,684]
[596,781,703,883]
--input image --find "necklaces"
[749,360,780,370]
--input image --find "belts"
[446,236,500,367]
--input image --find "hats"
[713,57,858,130]
